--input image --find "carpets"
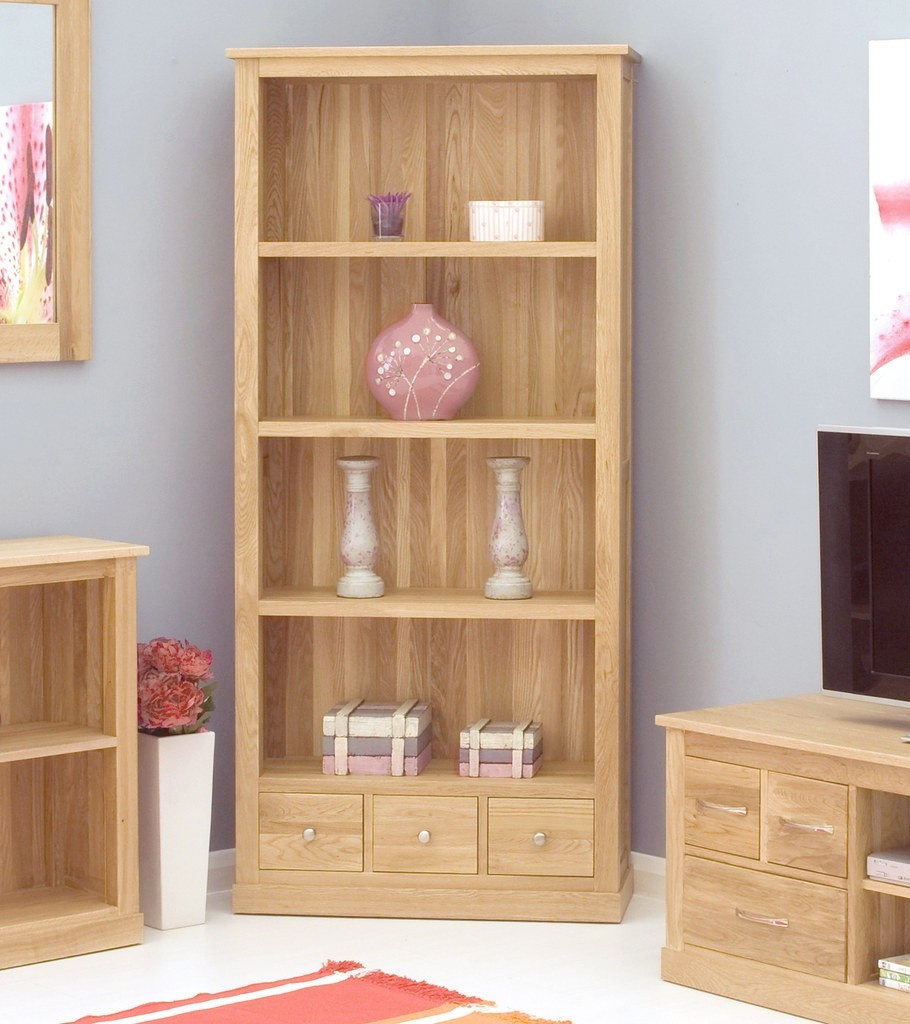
[57,959,571,1024]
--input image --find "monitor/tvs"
[816,425,910,707]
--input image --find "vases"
[366,304,480,421]
[138,729,216,931]
[485,457,533,597]
[335,455,384,599]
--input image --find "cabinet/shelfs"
[0,538,149,972]
[225,44,642,924]
[654,696,910,1024]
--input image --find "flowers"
[137,637,221,735]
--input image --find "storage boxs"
[467,200,545,243]
[322,699,432,776]
[459,719,544,777]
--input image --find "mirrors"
[0,0,93,364]
